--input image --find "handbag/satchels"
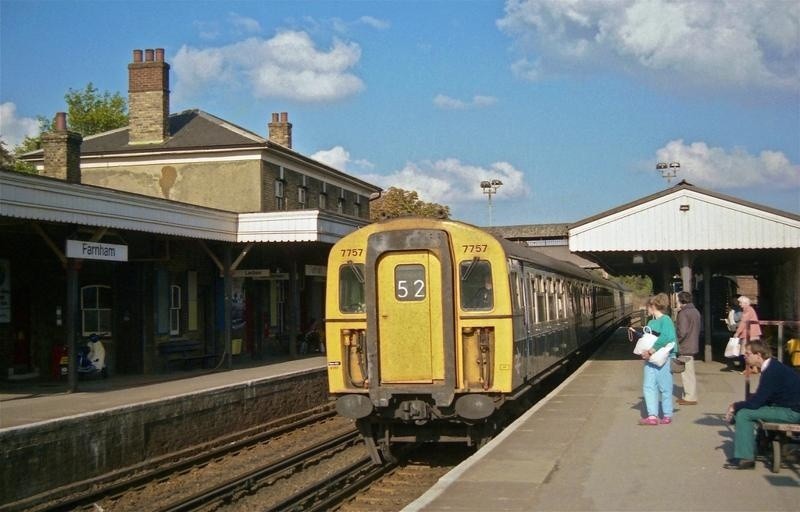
[725,337,740,358]
[633,333,675,367]
[670,357,690,373]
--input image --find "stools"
[759,420,800,473]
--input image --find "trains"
[323,217,636,466]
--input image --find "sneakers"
[724,457,755,469]
[640,418,657,425]
[659,416,672,424]
[676,398,697,405]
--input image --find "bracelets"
[647,350,652,356]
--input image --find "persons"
[721,294,744,372]
[731,293,762,375]
[721,339,799,469]
[673,291,703,407]
[637,293,680,426]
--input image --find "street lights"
[480,179,503,225]
[656,161,681,189]
